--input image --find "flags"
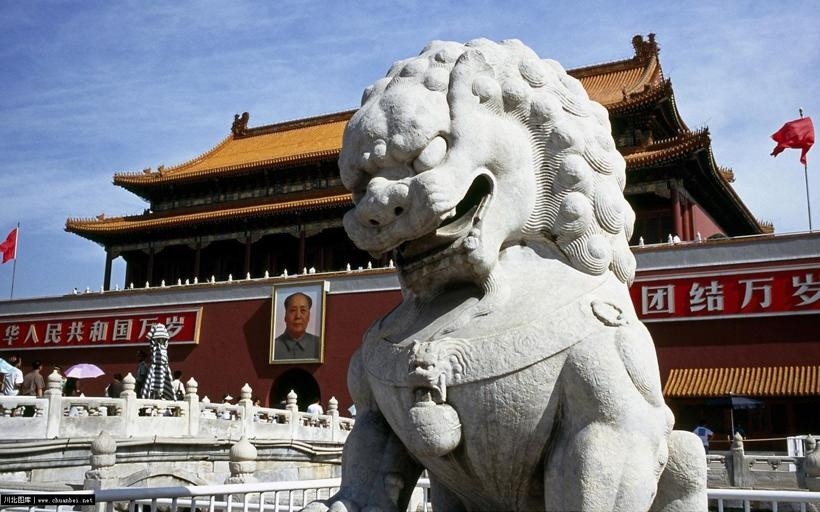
[770,116,815,165]
[0,227,18,264]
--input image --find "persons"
[0,353,323,417]
[692,421,715,454]
[275,293,320,359]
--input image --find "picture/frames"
[269,280,326,365]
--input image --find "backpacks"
[176,383,185,400]
